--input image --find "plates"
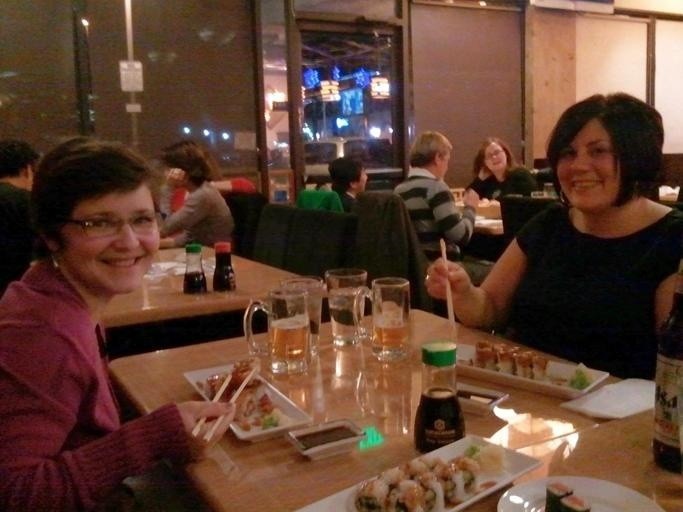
[457,339,611,400]
[184,362,313,444]
[291,431,538,511]
[287,417,364,460]
[499,475,663,512]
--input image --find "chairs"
[296,190,344,213]
[248,203,297,269]
[283,209,359,281]
[351,191,442,316]
[501,197,567,246]
[221,190,269,260]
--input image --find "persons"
[463,134,539,219]
[0,138,42,289]
[422,88,681,381]
[327,156,370,214]
[158,144,236,255]
[0,133,239,511]
[162,140,256,237]
[394,127,495,286]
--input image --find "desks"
[28,244,332,335]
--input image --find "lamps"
[369,41,390,99]
[319,54,341,102]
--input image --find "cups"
[235,266,408,372]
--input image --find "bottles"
[412,342,465,454]
[654,262,682,471]
[211,243,235,293]
[182,244,208,292]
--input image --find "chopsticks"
[190,373,256,445]
[434,236,460,348]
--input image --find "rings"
[425,274,430,280]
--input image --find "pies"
[207,355,267,422]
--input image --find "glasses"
[65,212,168,235]
[483,150,504,161]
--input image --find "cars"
[303,136,393,178]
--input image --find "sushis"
[354,457,481,512]
[544,480,591,511]
